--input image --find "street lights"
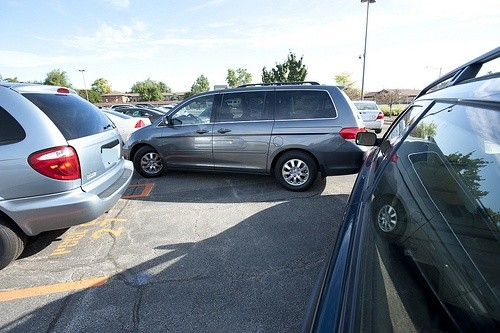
[361,0,376,100]
[79,69,89,102]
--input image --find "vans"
[368,139,499,332]
[123,80,372,191]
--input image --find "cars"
[300,44,500,332]
[0,81,135,270]
[110,104,188,123]
[351,100,384,133]
[99,108,152,143]
